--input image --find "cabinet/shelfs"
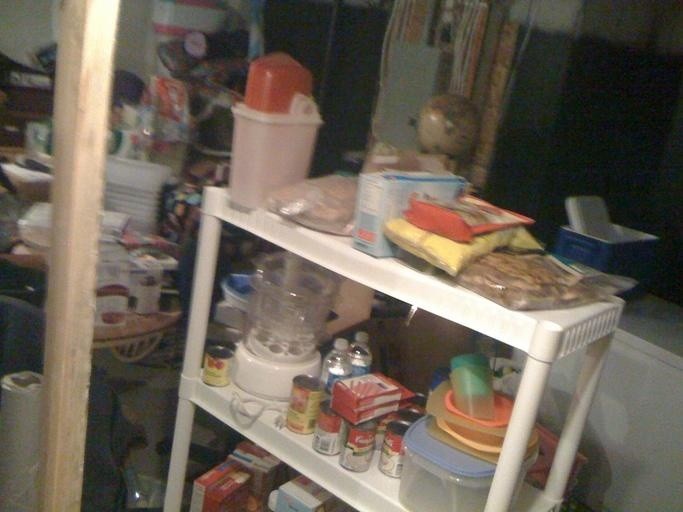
[163,185,627,512]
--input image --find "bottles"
[316,334,351,390]
[348,329,373,377]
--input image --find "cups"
[248,246,342,360]
[448,365,496,419]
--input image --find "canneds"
[284,375,327,434]
[311,400,348,457]
[202,345,235,388]
[340,420,377,474]
[378,420,412,478]
